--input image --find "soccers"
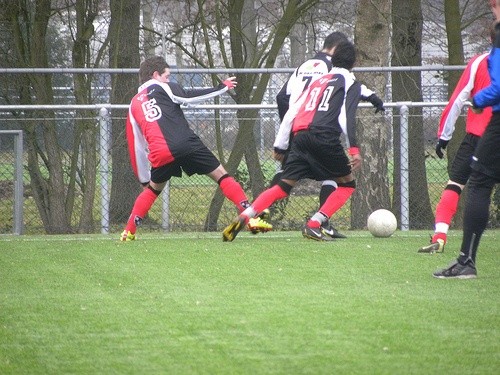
[367,209,397,237]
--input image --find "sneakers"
[248,217,272,232]
[432,262,478,279]
[320,222,347,238]
[222,219,241,241]
[302,224,336,241]
[120,230,135,241]
[417,234,444,253]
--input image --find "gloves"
[367,93,385,114]
[435,138,449,159]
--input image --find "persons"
[259,31,386,240]
[119,56,274,240]
[418,20,499,253]
[433,0,499,280]
[220,40,363,242]
